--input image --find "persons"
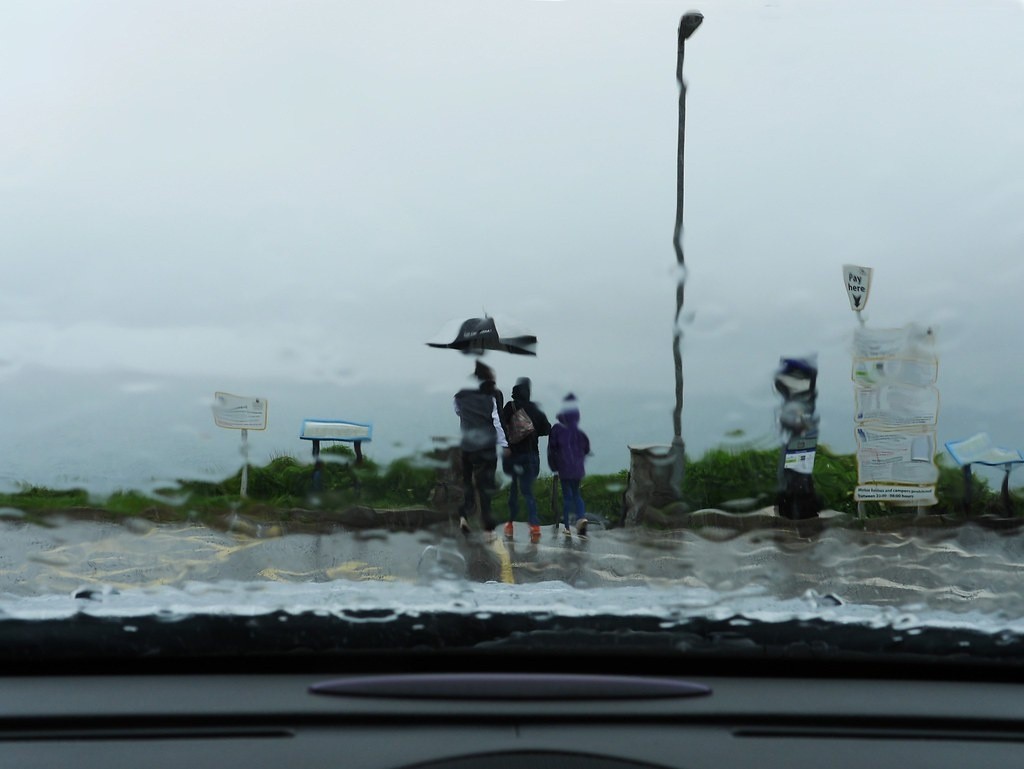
[548,391,591,535]
[449,359,509,534]
[502,376,555,541]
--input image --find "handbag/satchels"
[505,401,535,444]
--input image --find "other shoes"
[485,532,496,542]
[504,522,513,537]
[460,517,470,532]
[563,529,571,534]
[576,518,587,534]
[530,525,540,543]
